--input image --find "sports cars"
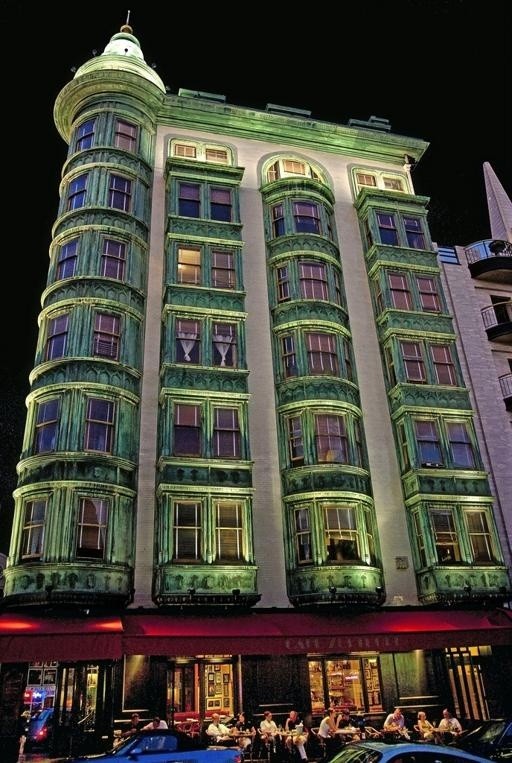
[82,728,244,763]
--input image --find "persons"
[417,710,443,744]
[438,706,464,745]
[258,709,280,756]
[284,709,311,761]
[235,710,257,754]
[139,715,169,730]
[120,711,143,738]
[338,710,361,744]
[206,711,237,747]
[383,706,406,744]
[318,706,344,757]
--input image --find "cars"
[449,718,512,763]
[324,737,496,762]
